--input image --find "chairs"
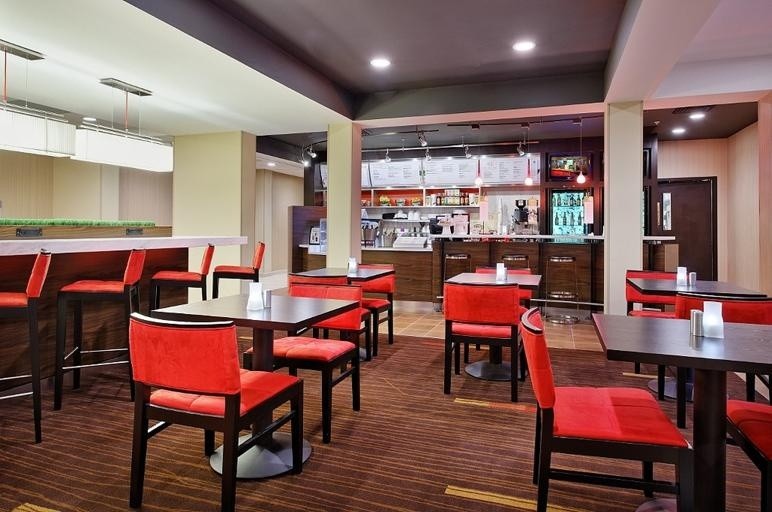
[351,263,395,357]
[52,248,146,411]
[657,292,772,429]
[520,306,694,512]
[625,269,679,373]
[443,281,527,403]
[0,247,52,442]
[127,313,303,511]
[476,266,532,309]
[242,282,362,445]
[149,242,214,310]
[211,240,266,298]
[288,273,371,374]
[725,400,772,512]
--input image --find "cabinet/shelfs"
[431,233,605,311]
[298,243,433,302]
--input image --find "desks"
[627,278,768,403]
[150,295,359,482]
[446,273,542,380]
[590,311,772,512]
[0,236,249,256]
[289,267,395,362]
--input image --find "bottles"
[464,192,468,206]
[570,212,574,226]
[576,195,581,207]
[577,212,581,225]
[460,192,464,206]
[557,193,561,206]
[569,193,574,206]
[563,212,567,225]
[436,193,441,206]
[555,212,560,225]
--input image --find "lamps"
[576,120,586,183]
[0,39,76,158]
[71,78,173,172]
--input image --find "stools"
[503,253,529,268]
[443,252,471,282]
[545,256,580,324]
